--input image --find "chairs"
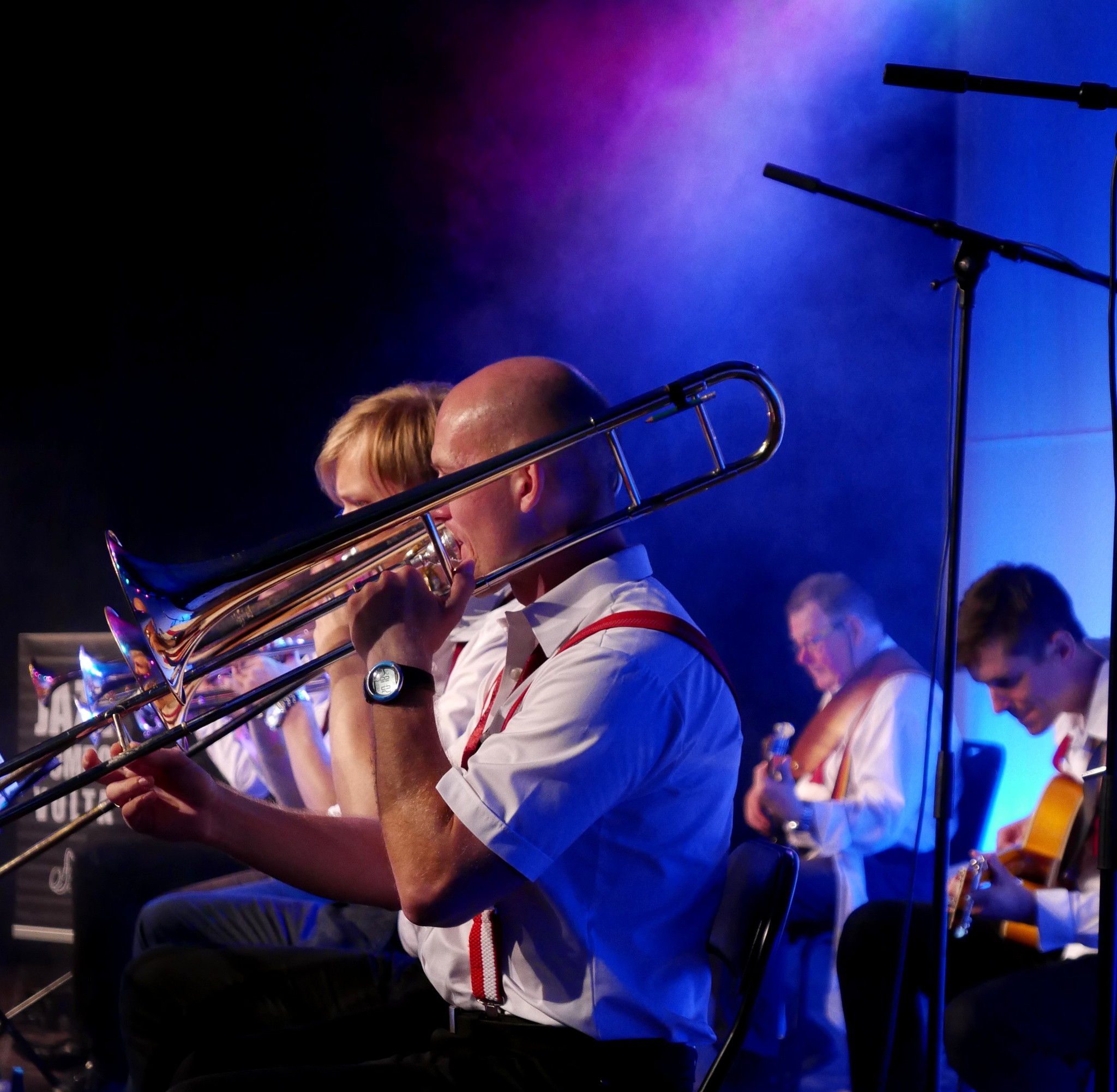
[785,740,1005,1078]
[694,840,800,1092]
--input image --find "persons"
[836,560,1109,1092]
[742,570,963,1092]
[65,360,739,1092]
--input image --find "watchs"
[263,690,312,729]
[364,660,437,704]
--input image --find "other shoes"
[14,1038,81,1070]
[53,1069,90,1092]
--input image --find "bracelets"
[794,803,816,834]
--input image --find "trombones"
[0,360,786,881]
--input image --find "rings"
[113,797,132,808]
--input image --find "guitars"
[761,724,820,862]
[949,775,1086,952]
